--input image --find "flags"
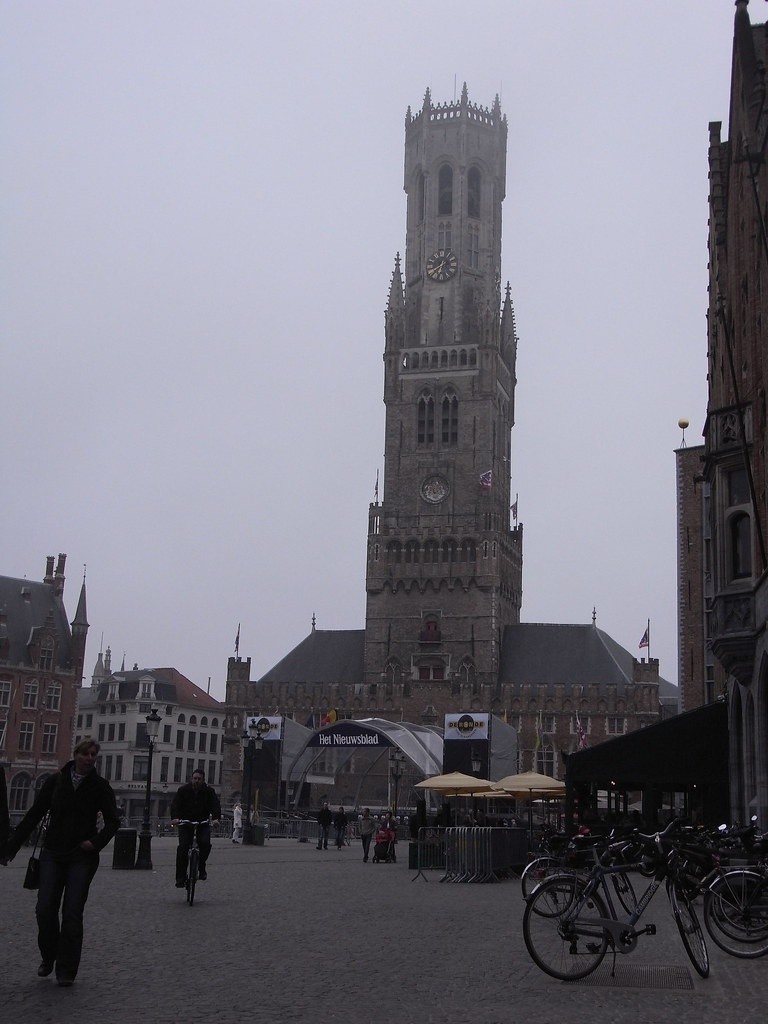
[511,502,516,518]
[577,720,585,750]
[320,709,336,725]
[480,470,492,487]
[639,629,648,648]
[234,635,239,652]
[305,712,313,728]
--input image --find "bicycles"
[172,819,219,906]
[522,814,711,982]
[519,807,768,960]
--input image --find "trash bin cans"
[252,824,266,847]
[112,828,138,870]
[408,842,420,869]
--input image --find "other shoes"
[324,847,327,849]
[199,865,208,880]
[60,982,72,987]
[316,846,321,850]
[176,877,185,887]
[38,956,54,977]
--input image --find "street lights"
[471,752,483,820]
[388,746,405,818]
[241,720,265,846]
[135,709,164,871]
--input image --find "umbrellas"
[414,770,567,848]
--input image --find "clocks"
[426,251,458,282]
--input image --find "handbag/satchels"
[22,857,40,889]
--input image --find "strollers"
[372,828,398,864]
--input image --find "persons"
[477,809,490,828]
[316,802,332,849]
[380,812,398,859]
[358,808,375,862]
[246,804,259,822]
[0,740,121,985]
[452,808,461,825]
[171,770,221,887]
[464,809,476,828]
[232,803,242,843]
[334,807,348,850]
